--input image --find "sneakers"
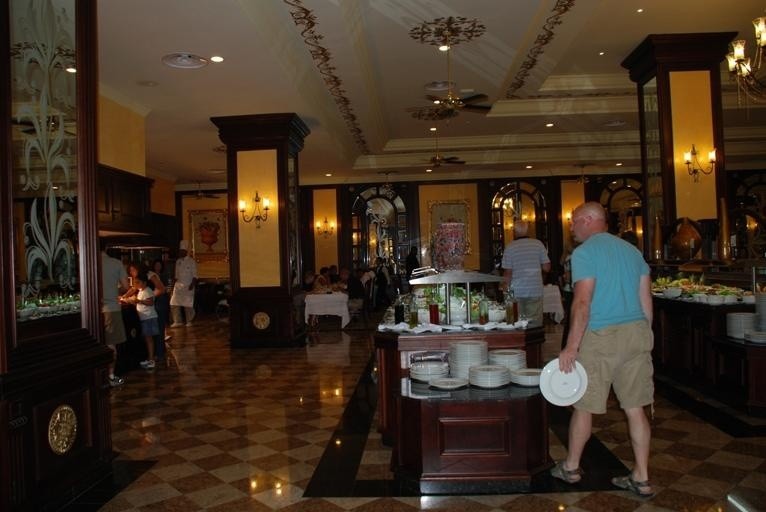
[185,322,192,327]
[140,360,149,365]
[142,361,156,368]
[108,375,125,386]
[170,322,184,328]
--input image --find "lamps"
[683,145,721,184]
[725,15,766,105]
[238,193,269,229]
[316,216,335,240]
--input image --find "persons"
[312,246,422,311]
[501,220,551,326]
[621,230,638,245]
[548,201,654,498]
[93,238,199,387]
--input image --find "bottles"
[394,289,519,326]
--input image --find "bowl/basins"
[413,288,506,325]
[689,294,755,306]
[662,288,682,298]
[17,300,80,318]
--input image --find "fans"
[404,29,491,120]
[409,126,466,169]
[181,178,220,201]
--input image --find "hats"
[179,240,188,250]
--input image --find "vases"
[434,223,467,270]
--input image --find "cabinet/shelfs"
[95,163,155,233]
[715,336,766,413]
[653,297,756,392]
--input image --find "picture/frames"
[426,198,473,256]
[186,207,229,264]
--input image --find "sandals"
[611,475,655,497]
[551,459,584,483]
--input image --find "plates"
[409,340,543,390]
[540,359,588,407]
[725,293,766,343]
[411,388,540,398]
